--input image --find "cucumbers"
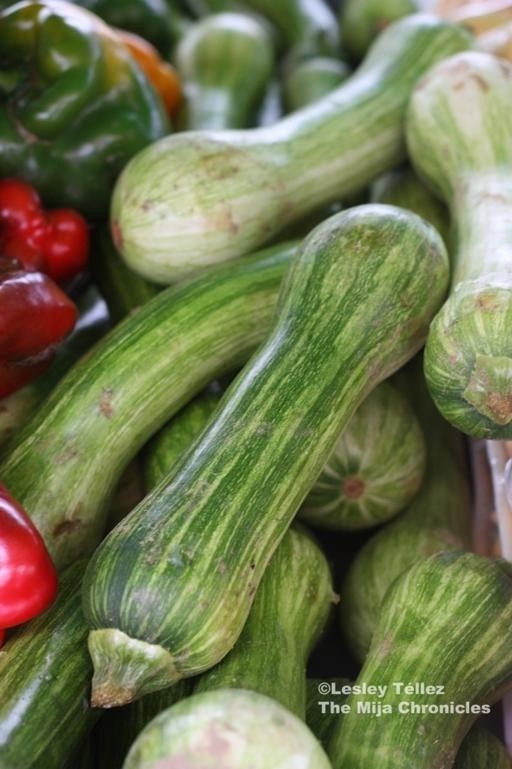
[0,0,511,766]
